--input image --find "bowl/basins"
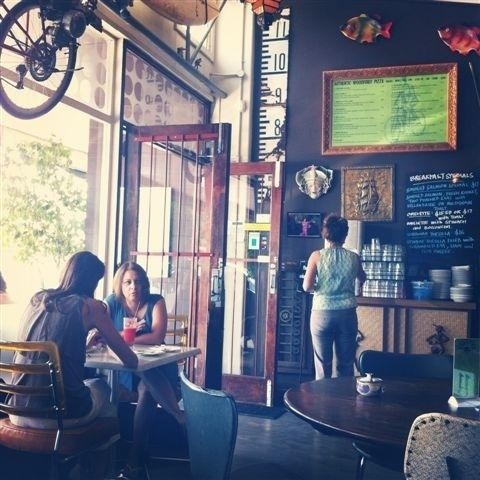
[409,264,475,304]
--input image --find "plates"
[151,345,181,353]
[134,348,165,356]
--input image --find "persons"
[301,213,367,380]
[92,260,185,480]
[4,250,139,480]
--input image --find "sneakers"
[114,465,151,480]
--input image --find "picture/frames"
[320,61,460,157]
[340,165,396,222]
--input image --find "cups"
[354,236,407,299]
[122,316,138,346]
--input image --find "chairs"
[0,313,192,480]
